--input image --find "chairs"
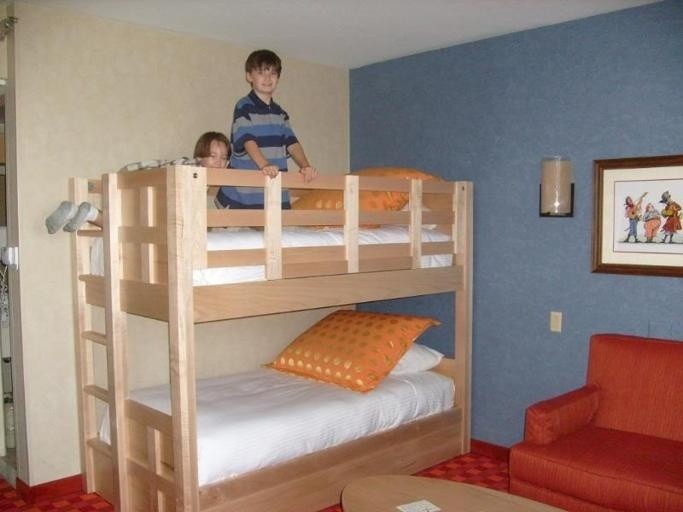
[506,334,683,511]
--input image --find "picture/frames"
[590,155,683,278]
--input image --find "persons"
[215,46,320,233]
[621,196,643,243]
[659,190,681,243]
[44,130,235,234]
[642,202,660,243]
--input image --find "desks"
[342,475,566,512]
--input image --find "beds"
[69,164,473,511]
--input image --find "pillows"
[262,309,442,394]
[290,168,435,229]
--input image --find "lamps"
[539,155,574,218]
